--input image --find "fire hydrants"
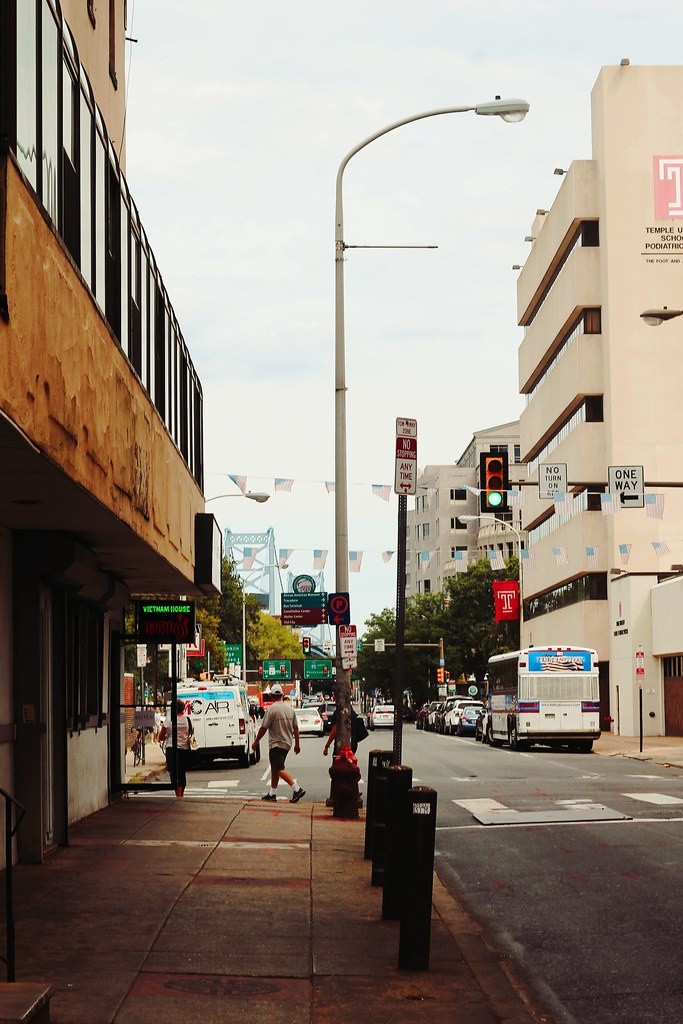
[328,747,361,820]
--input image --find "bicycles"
[129,726,150,766]
[159,735,167,754]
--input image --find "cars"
[367,704,394,730]
[475,707,489,743]
[456,706,482,736]
[294,709,324,737]
[317,702,337,732]
[302,696,323,710]
[416,695,484,734]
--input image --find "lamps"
[525,237,536,242]
[671,565,683,573]
[536,210,549,215]
[610,568,627,574]
[620,58,629,68]
[554,168,567,175]
[513,265,523,269]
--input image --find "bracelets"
[325,745,330,748]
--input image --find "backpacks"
[249,703,258,716]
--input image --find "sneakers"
[289,788,306,803]
[261,792,277,801]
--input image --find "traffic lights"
[437,668,444,682]
[303,637,311,655]
[281,665,285,676]
[479,451,509,513]
[258,666,262,676]
[445,671,449,682]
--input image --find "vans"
[176,675,260,766]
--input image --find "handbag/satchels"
[187,716,200,751]
[351,716,369,745]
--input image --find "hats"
[271,683,283,695]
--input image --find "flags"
[600,493,621,517]
[313,550,328,570]
[382,542,670,573]
[349,551,363,572]
[279,549,294,571]
[460,484,481,496]
[274,478,294,493]
[227,475,247,494]
[325,482,335,495]
[372,484,391,502]
[553,492,574,515]
[242,547,258,569]
[507,490,525,509]
[645,494,665,519]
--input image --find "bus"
[259,693,284,712]
[485,645,602,752]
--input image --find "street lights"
[325,94,529,813]
[458,515,524,647]
[242,565,289,682]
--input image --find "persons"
[252,684,306,803]
[323,691,363,798]
[159,700,194,798]
[144,706,162,745]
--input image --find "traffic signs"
[304,659,332,679]
[221,645,242,666]
[608,465,645,509]
[262,660,292,680]
